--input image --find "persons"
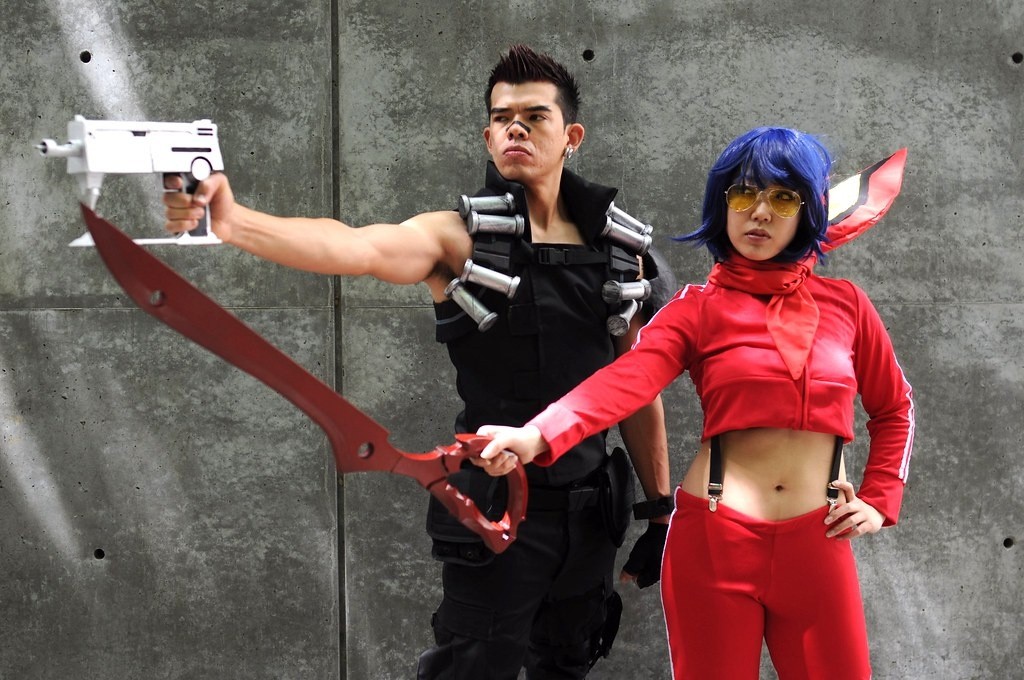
[161,44,675,680]
[464,126,917,680]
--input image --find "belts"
[497,487,605,510]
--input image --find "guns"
[32,116,228,248]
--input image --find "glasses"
[724,184,805,218]
